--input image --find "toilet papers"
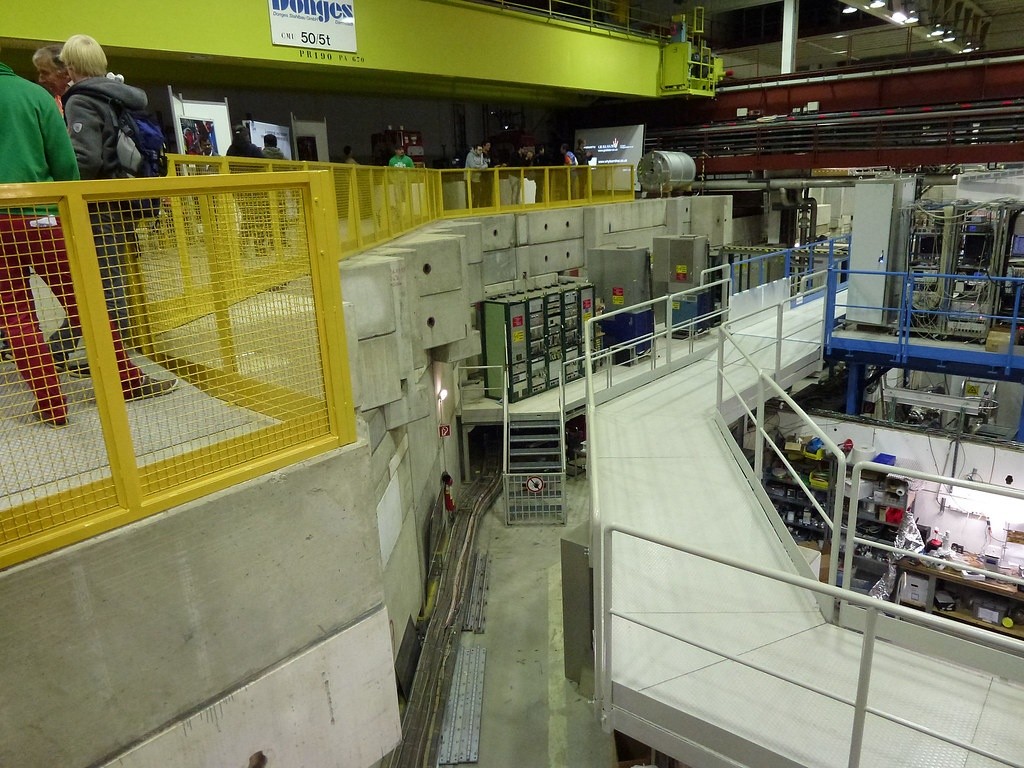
[851,444,876,466]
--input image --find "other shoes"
[6,353,66,372]
[68,363,90,378]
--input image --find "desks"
[898,554,1024,638]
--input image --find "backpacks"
[73,90,168,218]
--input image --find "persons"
[196,137,222,175]
[0,35,178,426]
[343,147,358,164]
[183,121,217,156]
[464,139,594,210]
[388,145,416,204]
[261,134,291,251]
[226,125,269,256]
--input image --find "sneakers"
[126,378,178,401]
[32,402,69,428]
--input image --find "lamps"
[842,5,858,14]
[869,0,974,53]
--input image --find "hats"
[562,144,569,150]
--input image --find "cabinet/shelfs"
[763,446,835,542]
[828,459,918,576]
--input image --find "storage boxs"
[802,446,826,460]
[844,480,873,500]
[873,453,897,466]
[985,327,1019,353]
[784,434,813,452]
[809,468,830,489]
[977,605,1005,624]
[899,572,929,603]
